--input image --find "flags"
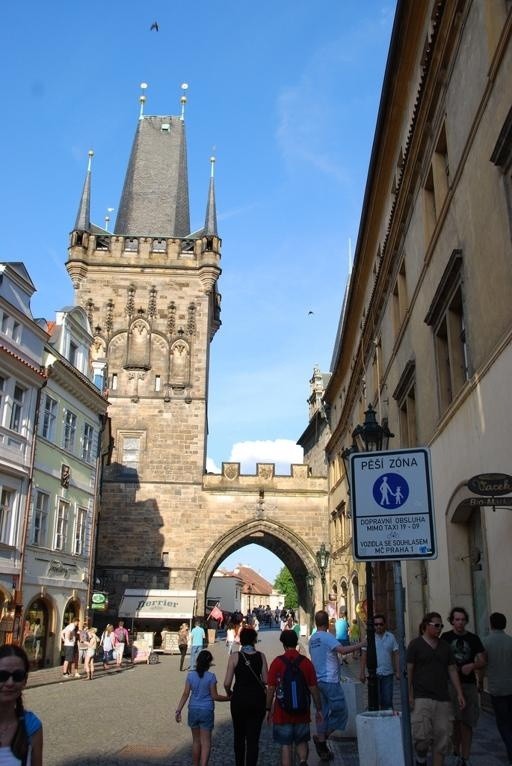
[225,621,247,655]
[211,606,223,627]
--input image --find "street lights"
[246,584,253,611]
[314,541,331,611]
[336,447,379,712]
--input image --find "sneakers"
[313,734,335,761]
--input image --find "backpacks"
[278,655,311,713]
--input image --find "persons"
[223,613,231,626]
[407,612,465,766]
[177,621,205,671]
[477,613,510,766]
[141,622,172,645]
[223,629,268,766]
[308,610,368,762]
[268,631,321,765]
[231,604,295,631]
[2,645,43,766]
[208,618,219,642]
[441,606,486,765]
[289,620,300,653]
[175,650,231,766]
[60,617,129,680]
[329,612,362,665]
[359,613,400,711]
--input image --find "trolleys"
[128,639,159,666]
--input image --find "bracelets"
[176,711,181,714]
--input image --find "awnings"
[118,596,196,618]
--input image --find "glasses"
[0,669,26,682]
[374,623,384,626]
[428,622,444,628]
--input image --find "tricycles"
[256,613,273,630]
